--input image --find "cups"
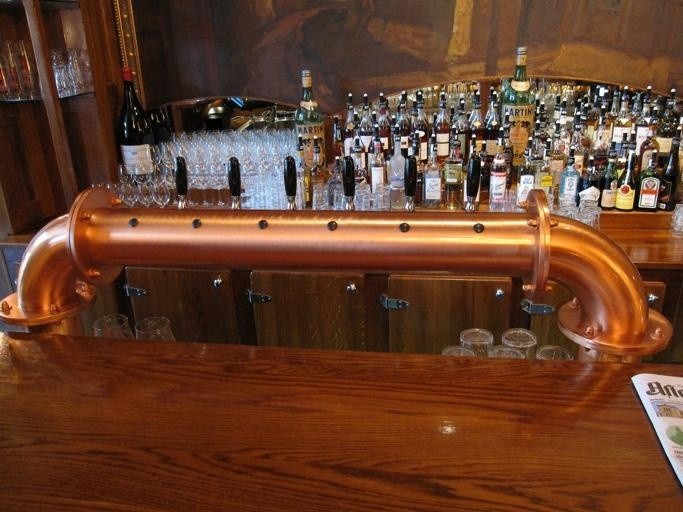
[437,324,580,361]
[89,313,176,343]
[669,203,683,235]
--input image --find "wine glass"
[112,125,305,213]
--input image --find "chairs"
[125,266,239,342]
[387,274,512,355]
[250,269,367,351]
[530,278,667,363]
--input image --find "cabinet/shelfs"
[0,2,125,246]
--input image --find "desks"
[0,332,680,511]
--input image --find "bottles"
[116,65,157,183]
[498,46,536,177]
[292,70,683,211]
[555,194,602,231]
[0,36,85,102]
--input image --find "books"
[629,371,683,484]
